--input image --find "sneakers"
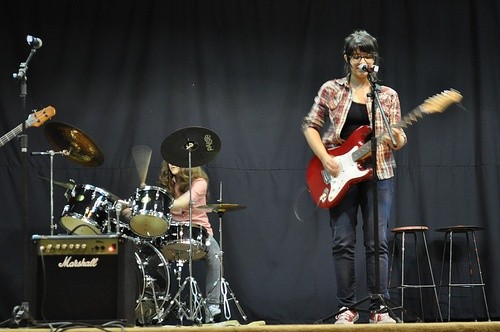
[369,310,397,323]
[334,307,360,325]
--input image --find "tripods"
[153,143,252,327]
[316,70,423,324]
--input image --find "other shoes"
[204,304,222,317]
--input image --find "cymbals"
[44,120,105,168]
[160,126,222,168]
[195,203,248,212]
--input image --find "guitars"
[305,88,464,210]
[0,103,56,148]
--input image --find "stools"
[434,225,491,323]
[388,225,444,323]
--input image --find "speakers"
[33,234,136,327]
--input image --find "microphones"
[27,35,42,48]
[359,64,371,72]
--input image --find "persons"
[303,31,408,326]
[118,159,223,327]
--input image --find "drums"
[133,240,172,321]
[127,185,175,238]
[58,183,117,235]
[159,222,209,262]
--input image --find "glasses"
[348,53,376,64]
